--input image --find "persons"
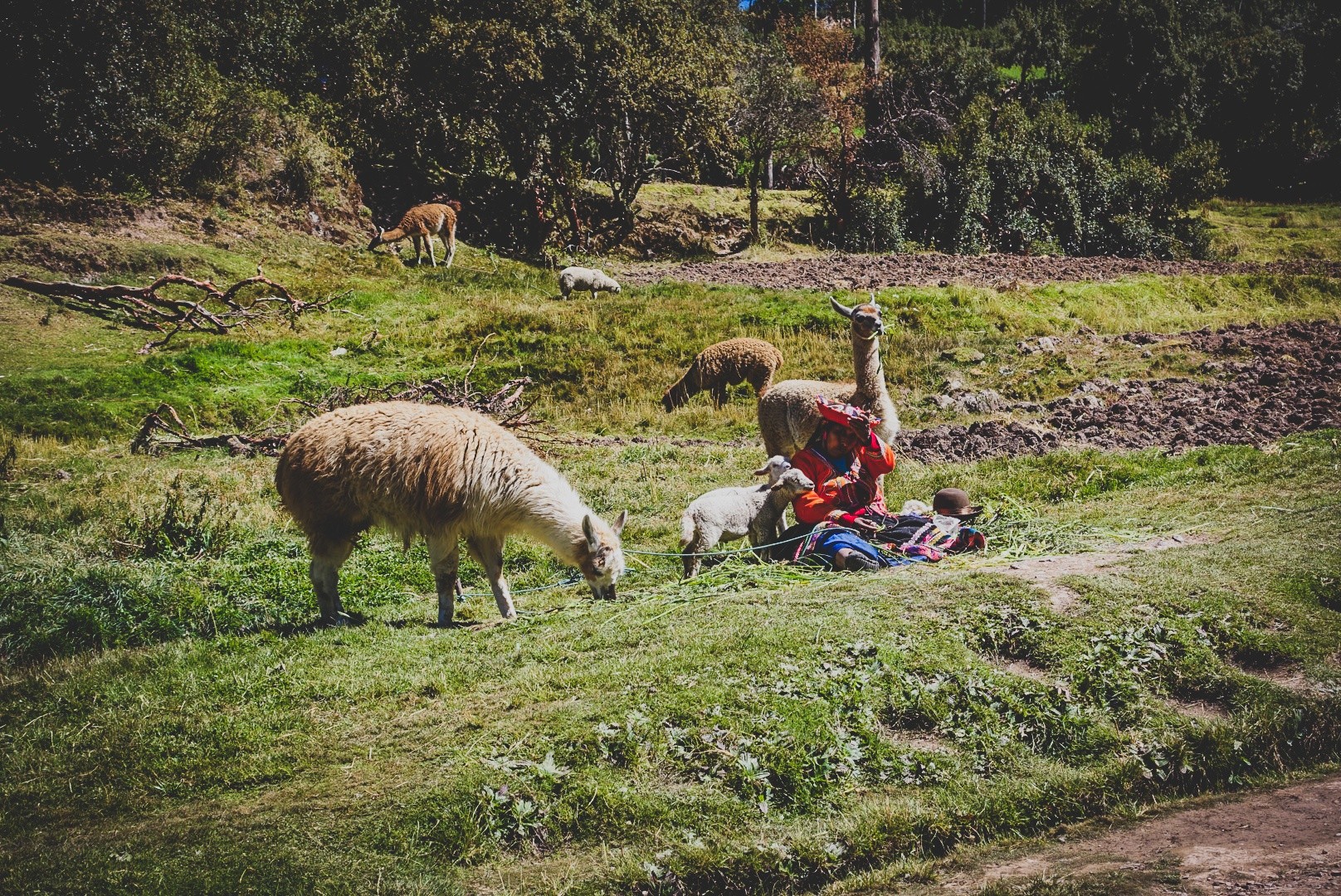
[770,395,987,571]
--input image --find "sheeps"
[368,200,460,268]
[679,455,816,578]
[661,338,783,415]
[758,296,900,536]
[560,266,621,301]
[277,402,625,621]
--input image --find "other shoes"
[845,552,880,572]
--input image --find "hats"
[816,395,881,428]
[931,487,984,519]
